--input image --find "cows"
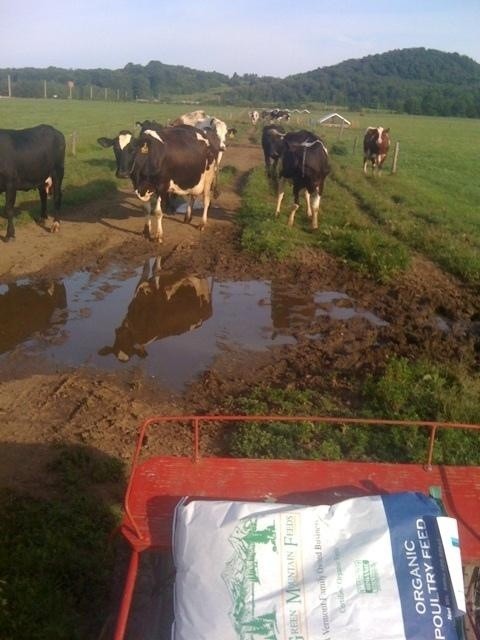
[91,256,215,363]
[95,108,237,242]
[261,122,332,231]
[247,109,290,127]
[0,123,67,244]
[362,125,392,176]
